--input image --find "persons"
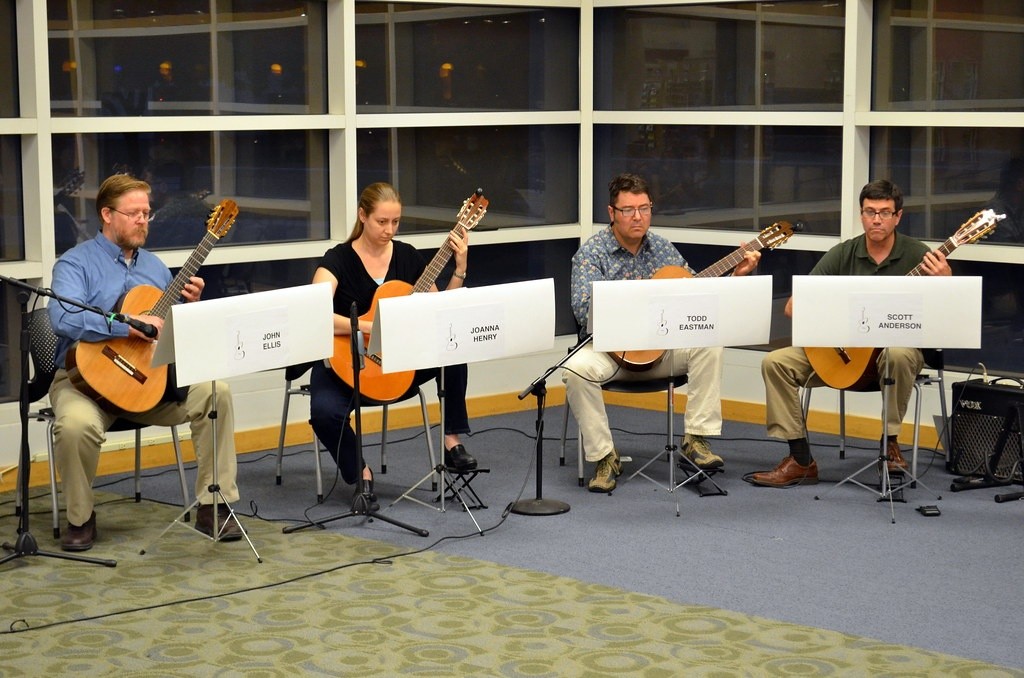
[571,175,762,492]
[742,179,953,487]
[311,183,477,514]
[47,174,248,551]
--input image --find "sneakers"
[195,504,243,541]
[60,510,97,550]
[678,432,725,469]
[588,448,624,493]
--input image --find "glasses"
[860,207,899,220]
[106,206,155,222]
[613,201,653,216]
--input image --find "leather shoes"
[878,438,908,472]
[741,454,819,487]
[444,443,477,470]
[351,465,374,513]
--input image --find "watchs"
[453,271,466,279]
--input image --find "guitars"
[607,221,795,372]
[68,197,242,419]
[801,208,1007,389]
[328,187,490,402]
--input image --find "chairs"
[275,359,439,503]
[803,347,952,497]
[558,303,687,486]
[21,308,191,539]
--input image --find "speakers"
[949,378,1024,485]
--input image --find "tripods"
[0,275,118,567]
[793,275,981,522]
[139,278,556,564]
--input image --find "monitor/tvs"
[585,274,773,516]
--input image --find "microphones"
[115,313,158,338]
